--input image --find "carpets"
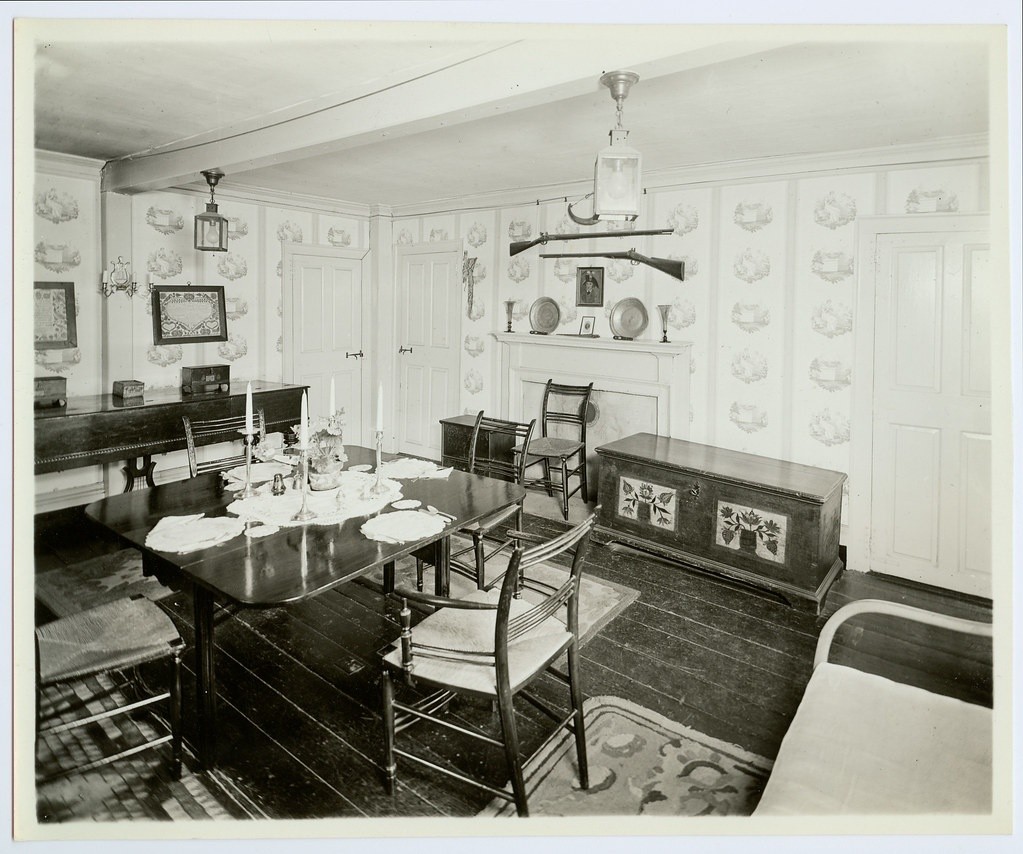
[36,675,274,822]
[475,695,775,816]
[35,548,180,619]
[362,533,641,668]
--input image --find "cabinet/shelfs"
[439,415,517,484]
[594,432,848,616]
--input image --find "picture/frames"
[151,285,228,345]
[576,266,604,307]
[33,281,77,350]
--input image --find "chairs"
[377,379,603,818]
[35,594,187,787]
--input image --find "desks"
[84,446,526,771]
[34,380,311,493]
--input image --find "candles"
[330,377,336,417]
[301,389,308,446]
[377,383,383,432]
[246,382,253,430]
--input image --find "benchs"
[750,599,993,815]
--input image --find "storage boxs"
[113,380,145,397]
[183,364,230,394]
[34,376,67,407]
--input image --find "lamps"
[593,70,642,223]
[102,256,156,297]
[194,168,228,252]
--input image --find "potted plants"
[290,406,348,490]
